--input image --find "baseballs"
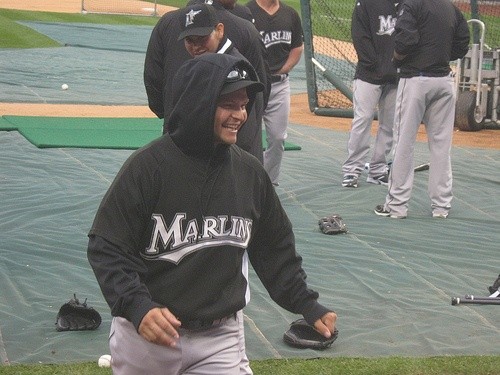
[61,83,70,91]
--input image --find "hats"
[177,4,221,43]
[220,68,265,96]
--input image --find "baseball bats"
[450,294,500,306]
[414,164,429,173]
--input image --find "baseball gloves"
[318,216,347,237]
[283,318,339,348]
[55,299,103,333]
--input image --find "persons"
[342,0,400,188]
[86,53,337,375]
[374,0,470,219]
[175,4,266,375]
[144,0,304,188]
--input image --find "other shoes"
[342,174,358,188]
[374,205,407,218]
[367,174,389,185]
